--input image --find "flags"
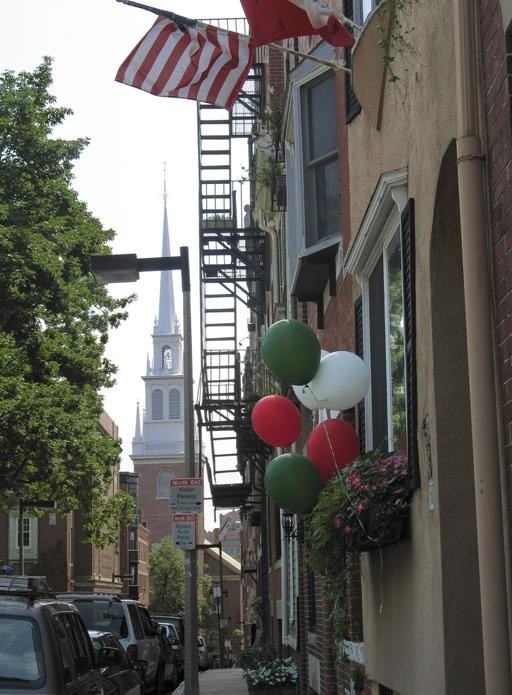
[115,20,254,113]
[241,0,357,49]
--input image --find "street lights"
[89,244,200,695]
[196,540,226,669]
[19,498,56,578]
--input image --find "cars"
[0,576,218,694]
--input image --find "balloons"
[248,319,370,517]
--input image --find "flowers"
[305,449,411,578]
